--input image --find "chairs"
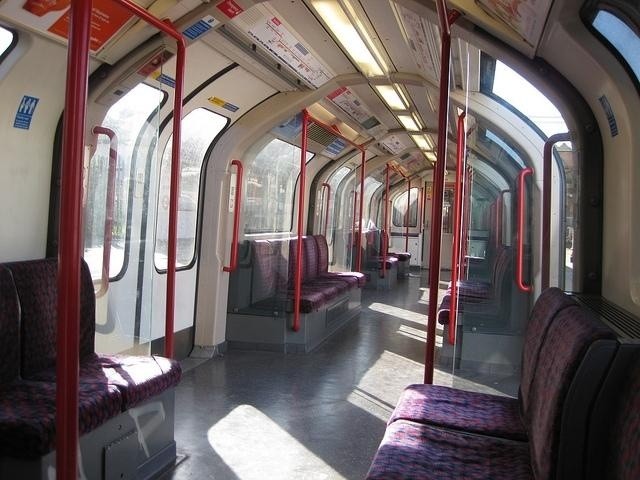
[226,228,412,353]
[438,244,532,377]
